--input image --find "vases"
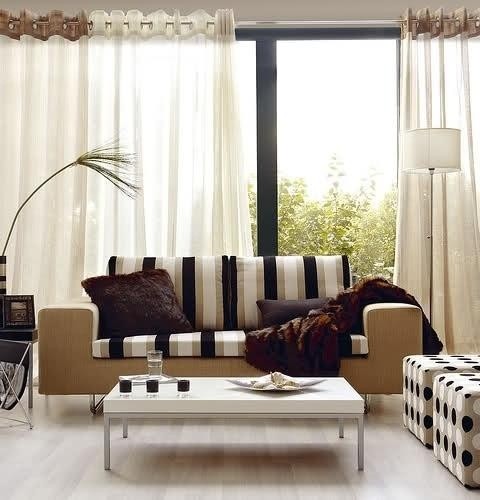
[0,254,8,296]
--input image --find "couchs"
[37,252,424,420]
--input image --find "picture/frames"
[2,294,36,329]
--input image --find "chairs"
[0,339,35,428]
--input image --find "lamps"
[401,126,463,336]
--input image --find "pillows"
[76,268,194,339]
[252,294,332,328]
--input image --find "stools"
[401,347,480,450]
[431,372,480,489]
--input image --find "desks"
[0,329,48,407]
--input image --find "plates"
[118,373,179,384]
[224,372,328,391]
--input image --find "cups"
[176,380,191,395]
[146,350,163,377]
[146,380,159,396]
[119,380,132,396]
